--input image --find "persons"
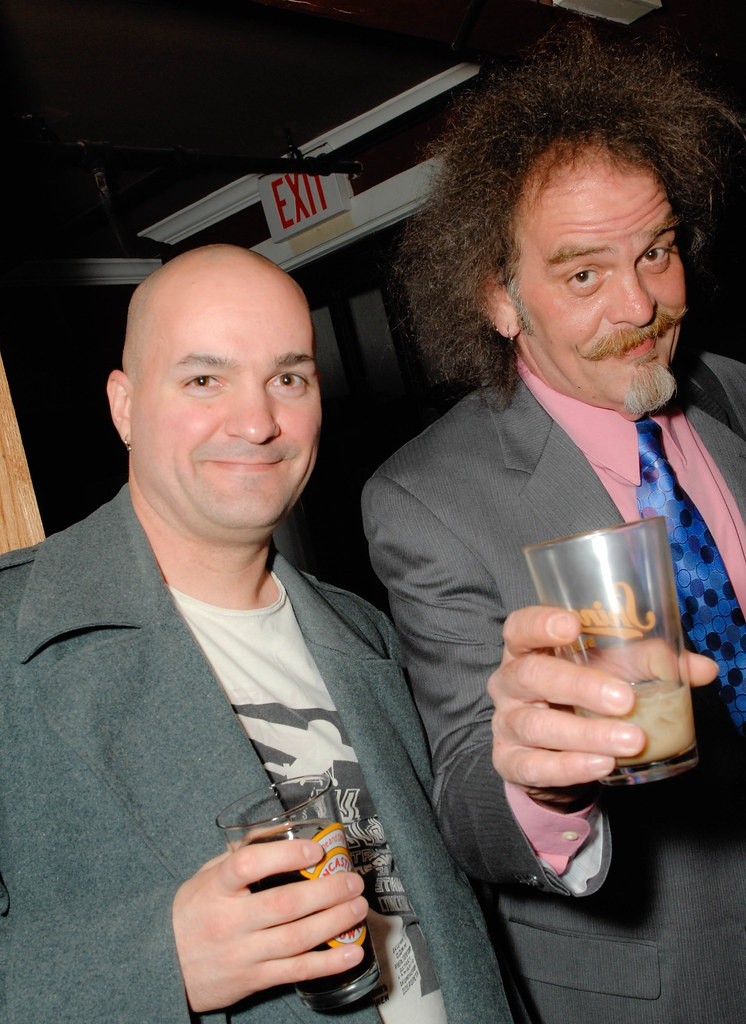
[2,246,534,1024]
[360,21,746,1024]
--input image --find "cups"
[522,516,699,784]
[214,774,380,1016]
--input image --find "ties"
[636,416,746,740]
